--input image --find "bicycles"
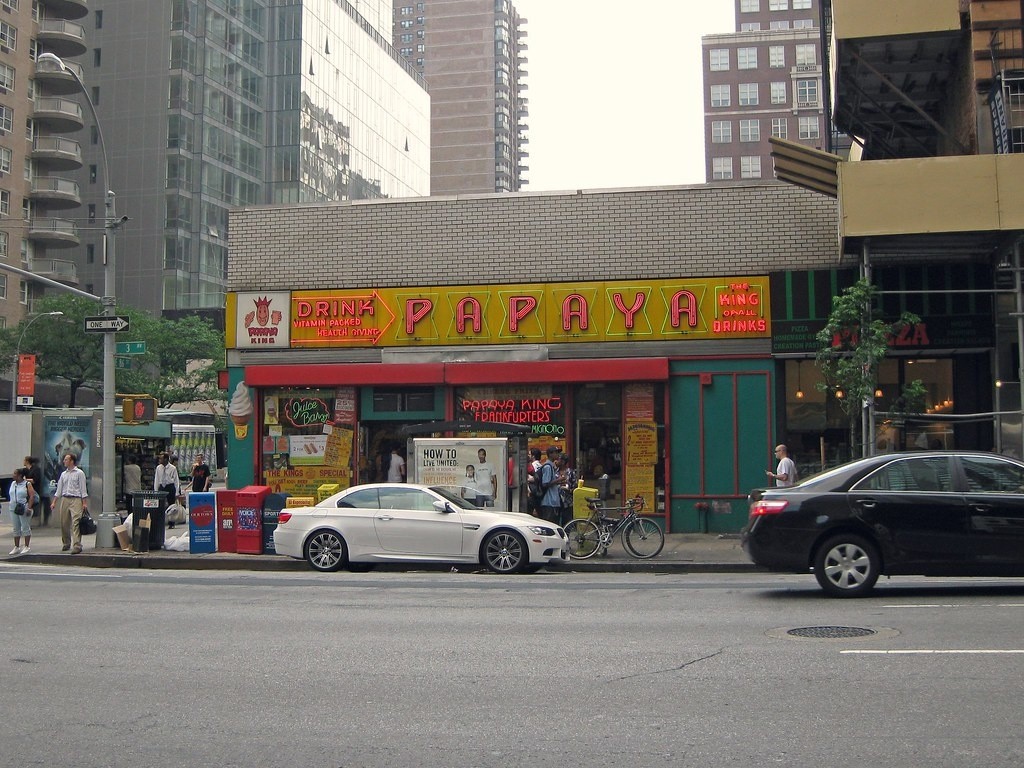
[561,494,665,560]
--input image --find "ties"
[162,468,166,487]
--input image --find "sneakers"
[9,546,21,555]
[21,546,30,553]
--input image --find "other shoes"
[71,547,82,554]
[62,545,69,551]
[169,523,174,529]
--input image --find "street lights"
[35,51,119,549]
[10,311,64,412]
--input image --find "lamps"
[796,360,804,399]
[835,386,843,398]
[875,387,883,398]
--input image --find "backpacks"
[529,463,553,500]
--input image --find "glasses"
[775,450,784,453]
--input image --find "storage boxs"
[317,483,339,503]
[134,526,149,552]
[285,497,314,509]
[111,525,130,550]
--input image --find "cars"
[273,481,572,575]
[738,447,1024,598]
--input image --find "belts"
[63,495,80,498]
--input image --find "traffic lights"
[132,398,158,422]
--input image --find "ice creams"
[229,381,253,426]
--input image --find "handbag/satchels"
[79,507,97,535]
[165,497,187,523]
[26,481,40,506]
[14,503,25,515]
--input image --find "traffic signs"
[83,315,130,335]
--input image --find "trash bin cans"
[130,490,169,552]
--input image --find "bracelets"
[772,474,775,477]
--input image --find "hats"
[546,446,563,456]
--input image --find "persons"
[8,468,36,554]
[123,455,142,516]
[460,464,478,508]
[764,443,800,488]
[189,453,212,492]
[527,447,544,518]
[22,455,40,519]
[50,453,89,553]
[154,452,179,529]
[381,444,405,483]
[473,448,498,507]
[168,447,178,463]
[540,446,571,527]
